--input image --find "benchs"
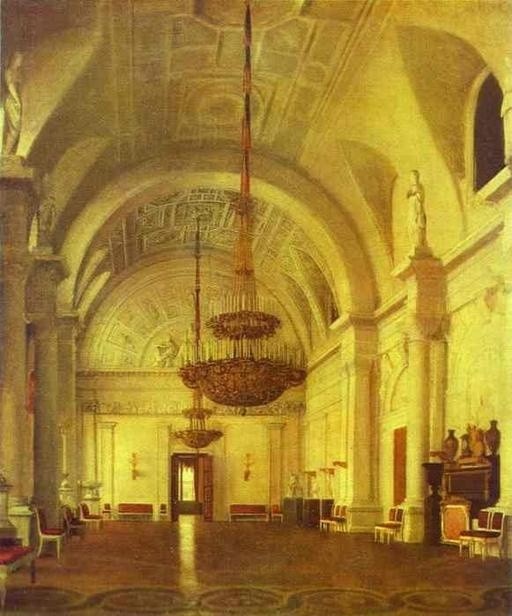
[117,503,152,523]
[226,503,269,523]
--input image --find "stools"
[0,543,37,609]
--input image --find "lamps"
[168,210,226,449]
[177,0,311,417]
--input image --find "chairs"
[160,503,169,520]
[34,503,114,559]
[319,504,345,537]
[373,507,406,546]
[271,504,283,522]
[457,508,506,567]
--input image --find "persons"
[0,47,25,158]
[407,168,430,250]
